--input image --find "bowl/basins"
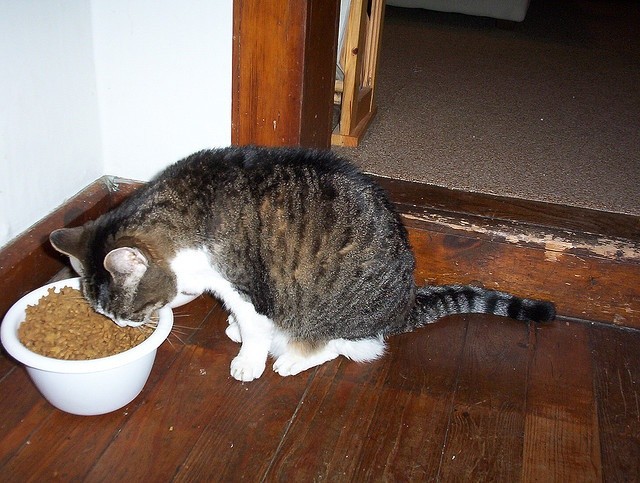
[0,275,174,417]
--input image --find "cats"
[47,146,552,382]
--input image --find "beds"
[385,0,529,31]
